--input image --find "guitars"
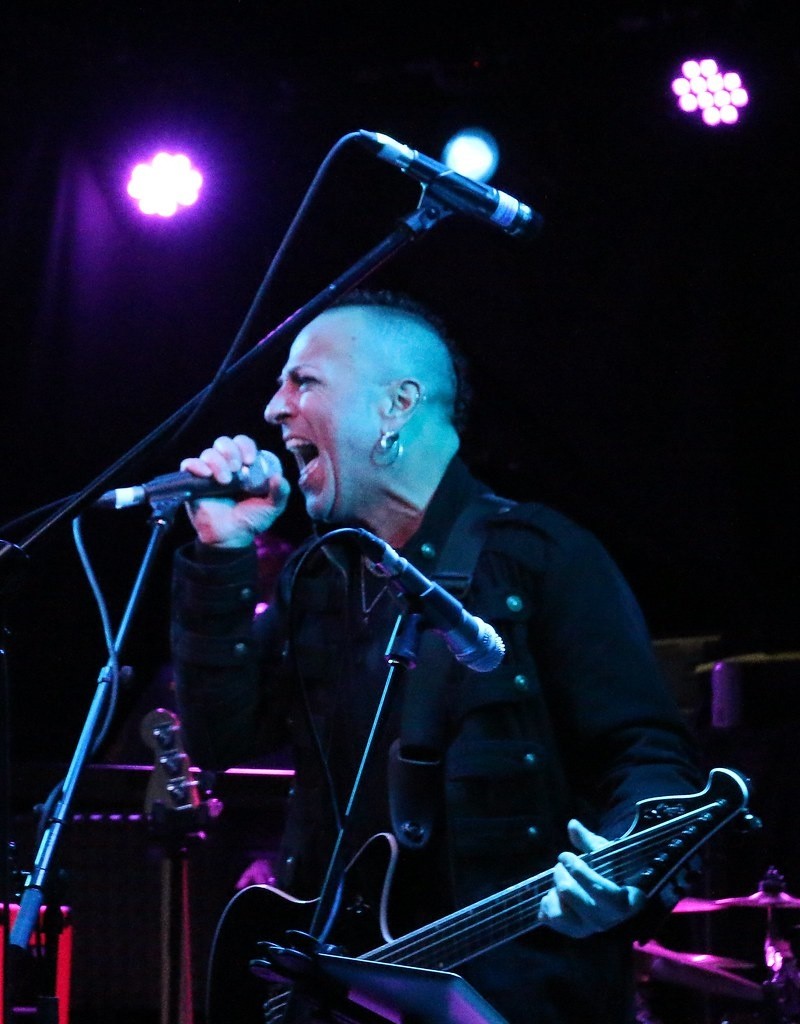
[198,766,755,1024]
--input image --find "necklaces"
[359,554,392,624]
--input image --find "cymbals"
[711,889,800,910]
[693,651,800,674]
[652,634,722,649]
[668,895,732,914]
[632,937,762,999]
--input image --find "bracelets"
[190,539,253,564]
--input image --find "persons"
[169,290,715,1024]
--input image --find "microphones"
[92,449,283,509]
[356,528,506,672]
[355,129,544,244]
